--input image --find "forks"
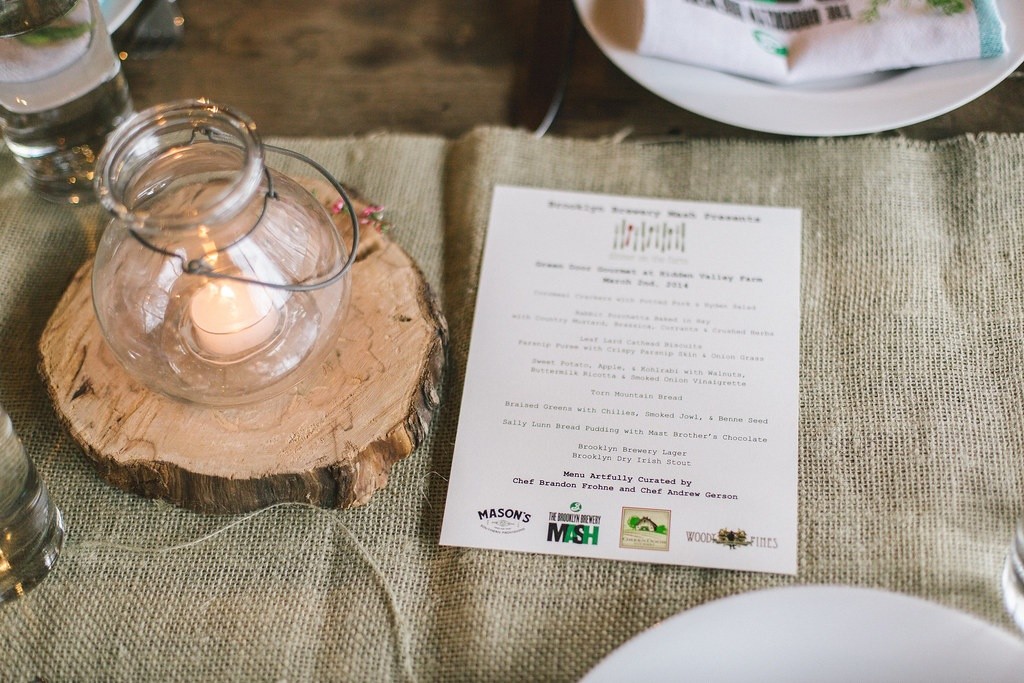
[126,0,185,65]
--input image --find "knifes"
[514,0,576,137]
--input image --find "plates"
[572,0,1024,137]
[578,585,1024,683]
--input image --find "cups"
[0,0,135,205]
[0,404,65,602]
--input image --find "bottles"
[90,99,350,405]
